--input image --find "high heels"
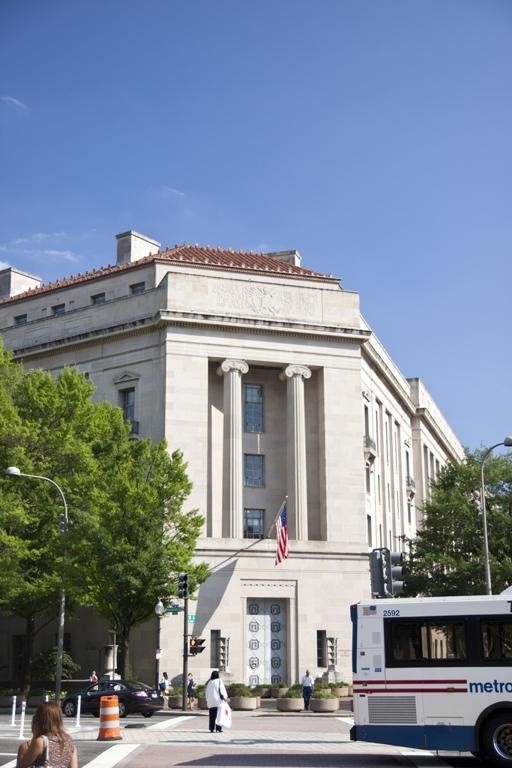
[210,729,223,732]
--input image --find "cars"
[60,678,166,718]
[4,687,58,708]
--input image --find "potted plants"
[168,679,354,711]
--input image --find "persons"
[89,670,98,690]
[159,672,170,694]
[17,702,78,768]
[17,715,36,768]
[187,673,197,711]
[205,670,228,732]
[301,669,314,710]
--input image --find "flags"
[273,504,288,567]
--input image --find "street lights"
[154,596,165,689]
[3,466,72,720]
[477,436,512,596]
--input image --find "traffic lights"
[189,637,205,656]
[176,572,188,598]
[389,548,407,597]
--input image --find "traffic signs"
[166,606,181,611]
[187,614,199,621]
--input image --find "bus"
[345,586,512,767]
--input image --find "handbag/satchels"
[215,699,232,728]
[159,683,166,691]
